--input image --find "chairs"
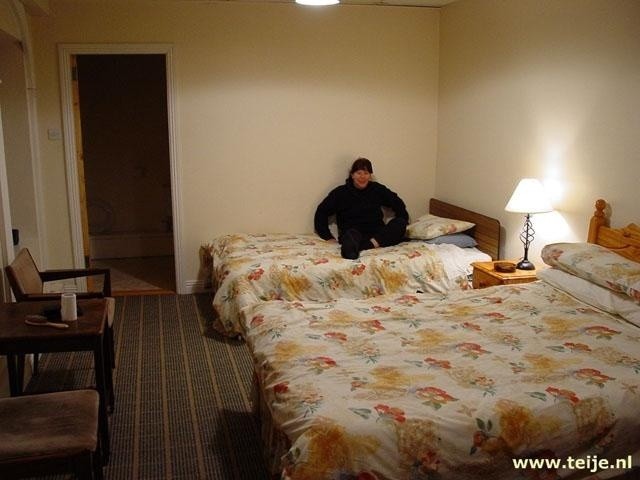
[0,248,116,480]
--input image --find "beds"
[238,200,640,480]
[202,198,500,340]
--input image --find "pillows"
[536,243,640,327]
[405,215,478,248]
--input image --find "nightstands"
[470,258,537,289]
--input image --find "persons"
[313,158,409,259]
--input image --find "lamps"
[505,179,553,269]
